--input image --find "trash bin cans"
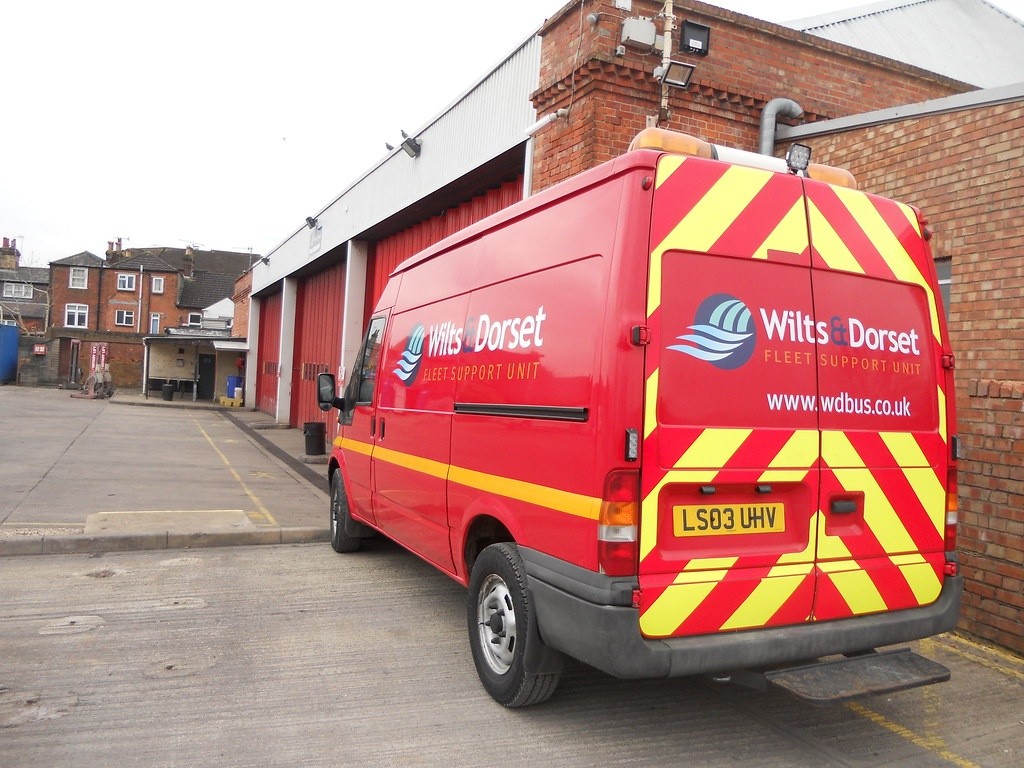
[162,384,174,401]
[304,422,326,455]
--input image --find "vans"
[317,127,960,707]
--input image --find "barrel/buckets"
[234,387,243,399]
[303,421,327,455]
[227,375,241,398]
[162,384,173,401]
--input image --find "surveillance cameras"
[524,113,557,137]
